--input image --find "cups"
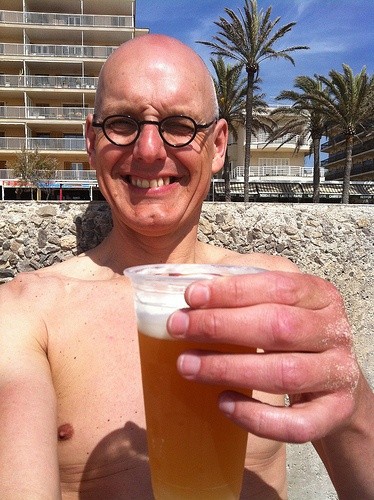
[123,262,272,500]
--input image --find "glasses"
[93,115,222,146]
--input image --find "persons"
[1,34,374,500]
